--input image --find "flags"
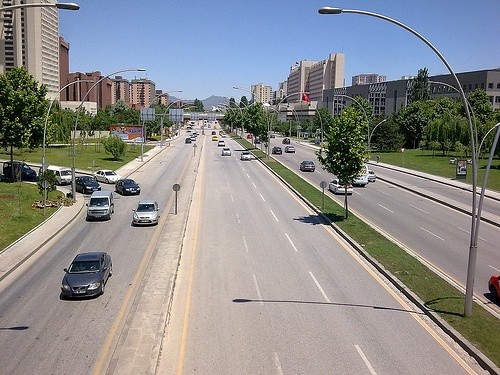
[302,93,310,102]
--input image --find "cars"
[61,252,113,297]
[132,200,160,225]
[75,176,102,194]
[85,191,115,221]
[488,274,500,302]
[368,171,376,183]
[115,179,141,196]
[93,170,122,184]
[328,179,353,195]
[185,121,295,161]
[2,161,36,181]
[300,161,315,172]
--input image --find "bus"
[338,162,368,187]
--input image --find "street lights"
[215,86,323,159]
[317,5,500,317]
[72,68,146,202]
[42,80,94,216]
[141,91,197,160]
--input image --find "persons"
[376,154,381,163]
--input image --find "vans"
[47,166,72,186]
